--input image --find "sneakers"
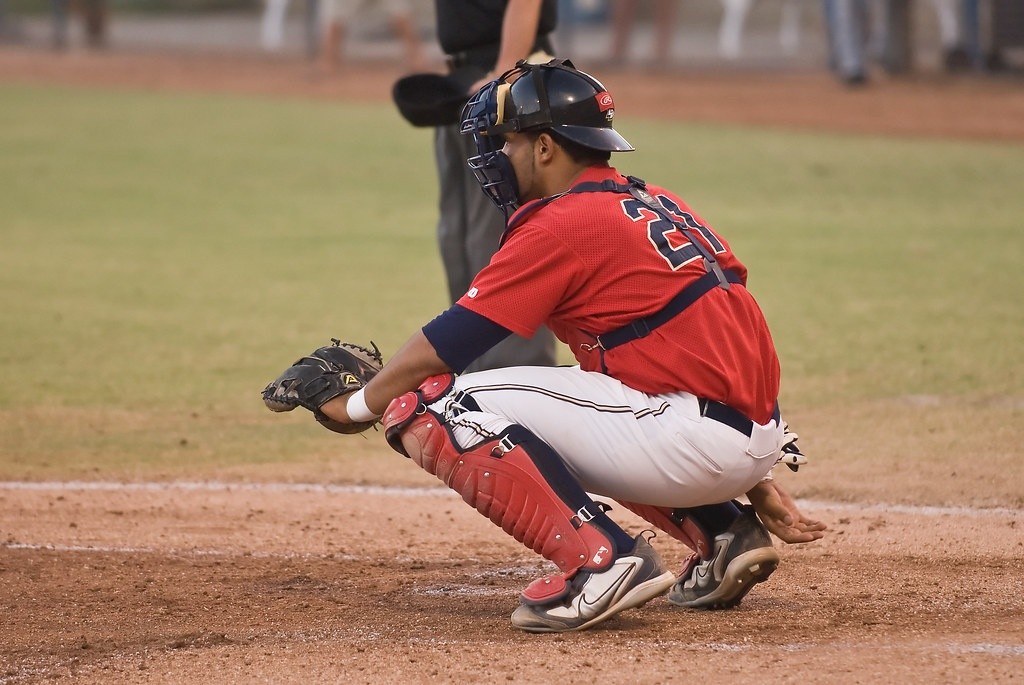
[669,503,779,608]
[510,529,675,633]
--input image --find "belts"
[697,397,781,437]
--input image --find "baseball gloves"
[259,344,384,435]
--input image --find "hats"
[393,74,472,127]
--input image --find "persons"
[430,0,558,377]
[262,55,827,636]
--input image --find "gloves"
[775,421,808,473]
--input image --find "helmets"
[507,61,636,152]
